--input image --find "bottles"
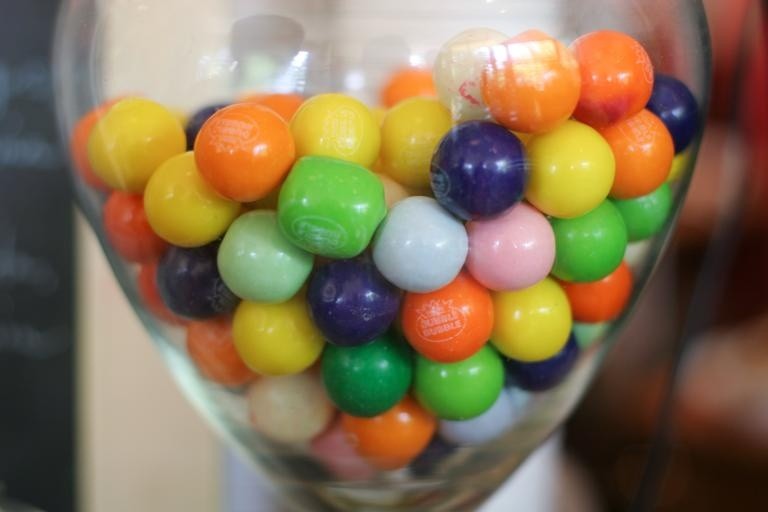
[49,0,711,511]
